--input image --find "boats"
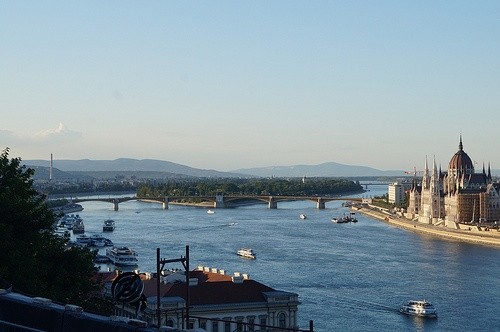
[52,213,114,263]
[330,213,358,223]
[102,217,116,232]
[106,245,139,267]
[300,214,306,219]
[236,248,256,259]
[400,300,439,318]
[207,210,214,214]
